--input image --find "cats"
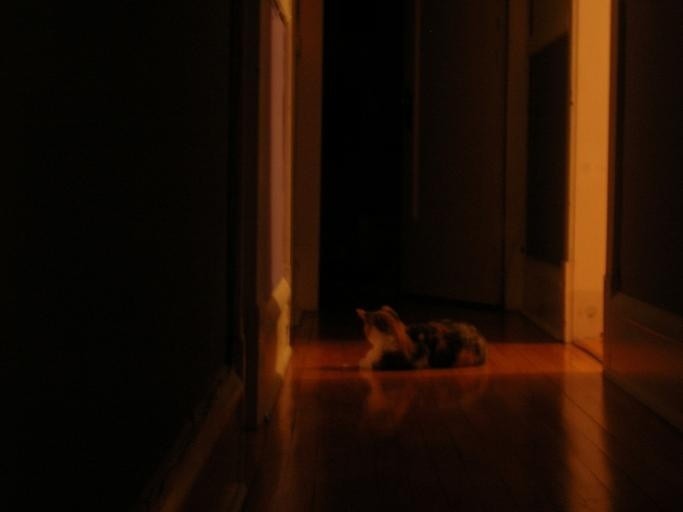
[354,302,486,371]
